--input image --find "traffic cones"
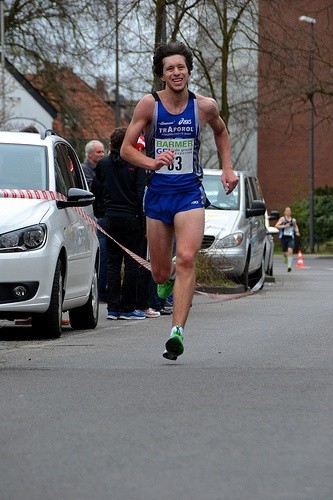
[296,250,305,268]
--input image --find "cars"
[173,168,280,290]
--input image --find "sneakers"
[157,256,177,299]
[163,325,184,361]
[106,311,146,319]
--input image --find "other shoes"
[135,308,160,317]
[164,301,172,307]
[155,308,172,315]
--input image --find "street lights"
[298,16,316,254]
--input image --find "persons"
[276,207,300,271]
[119,41,240,361]
[81,129,174,321]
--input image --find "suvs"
[0,129,101,339]
